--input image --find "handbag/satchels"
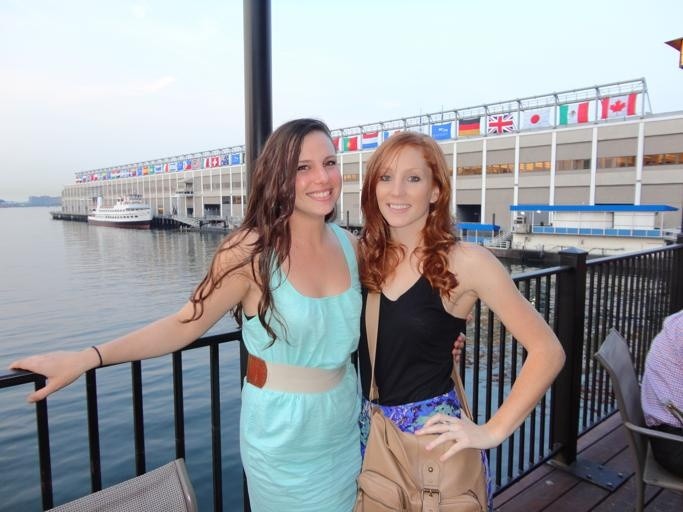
[354,407,489,512]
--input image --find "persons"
[642,309,683,479]
[353,131,566,512]
[10,119,465,512]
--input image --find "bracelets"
[91,346,102,367]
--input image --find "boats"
[87,191,155,231]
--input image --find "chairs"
[594,327,681,510]
[40,457,198,512]
